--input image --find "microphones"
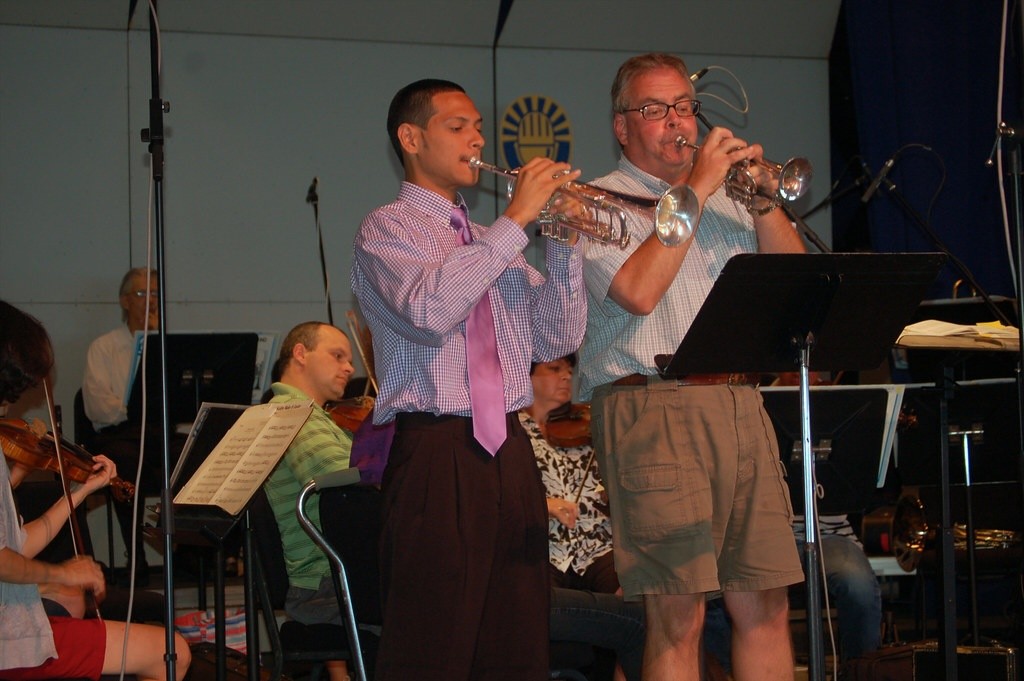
[860,157,884,196]
[862,157,896,203]
[306,177,318,203]
[690,68,709,82]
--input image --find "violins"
[543,400,595,451]
[323,394,380,432]
[0,417,137,502]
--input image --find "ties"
[450,208,507,458]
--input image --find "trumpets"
[467,154,701,255]
[673,133,816,209]
[890,494,1024,572]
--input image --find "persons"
[519,352,647,681]
[82,268,159,582]
[0,300,192,681]
[264,322,387,636]
[787,533,882,662]
[349,78,588,681]
[570,54,805,681]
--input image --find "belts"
[612,372,762,387]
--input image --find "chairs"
[74,386,159,588]
[248,468,381,681]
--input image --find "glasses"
[125,290,158,297]
[614,100,702,121]
